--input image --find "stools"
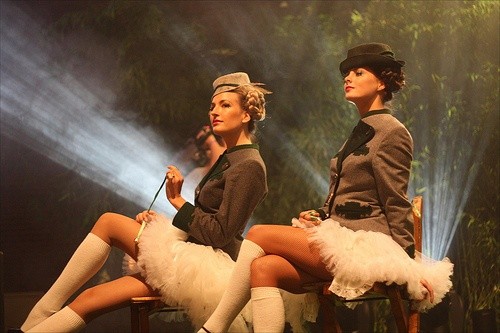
[130,297,184,333]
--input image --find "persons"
[195,44,454,333]
[0,71,319,333]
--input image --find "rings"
[172,173,174,176]
[303,214,307,219]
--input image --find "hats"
[211,71,266,98]
[339,43,405,73]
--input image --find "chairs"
[318,196,423,333]
